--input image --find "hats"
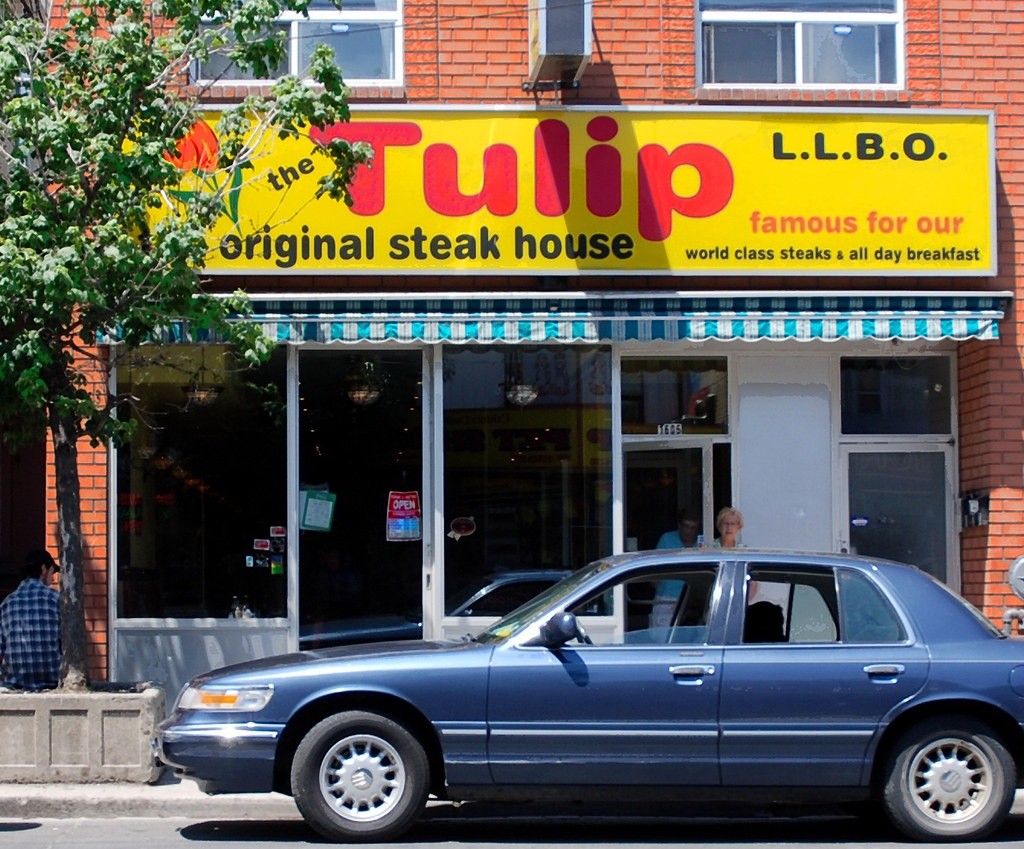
[25,549,60,574]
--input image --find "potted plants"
[0,0,375,782]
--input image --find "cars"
[298,566,606,648]
[149,548,1024,842]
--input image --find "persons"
[743,600,784,642]
[648,510,702,644]
[708,508,758,601]
[0,548,64,690]
